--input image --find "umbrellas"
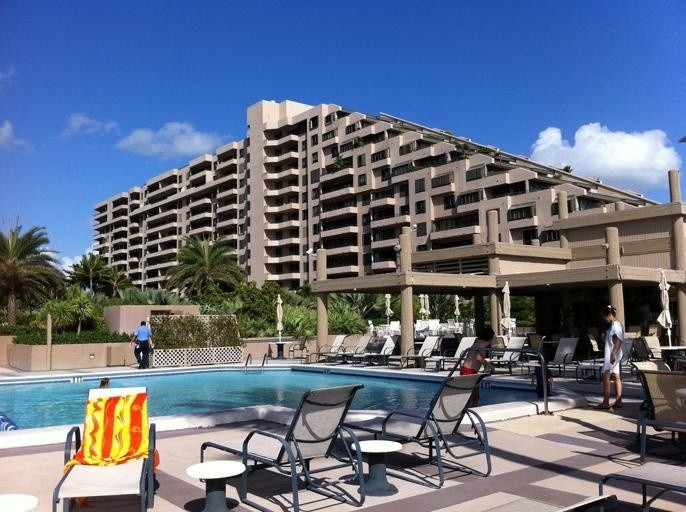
[454,295,460,322]
[384,294,393,327]
[274,294,284,341]
[656,271,673,346]
[502,281,515,341]
[419,293,425,321]
[424,294,430,319]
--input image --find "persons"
[459,326,496,407]
[531,357,554,397]
[594,304,624,409]
[128,320,154,369]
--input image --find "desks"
[351,438,403,496]
[659,346,686,371]
[267,342,292,360]
[186,461,246,512]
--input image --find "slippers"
[611,405,622,408]
[593,404,610,409]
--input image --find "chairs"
[51,386,155,512]
[200,384,366,512]
[329,374,495,488]
[287,335,308,359]
[584,324,665,367]
[631,359,685,464]
[309,333,582,379]
